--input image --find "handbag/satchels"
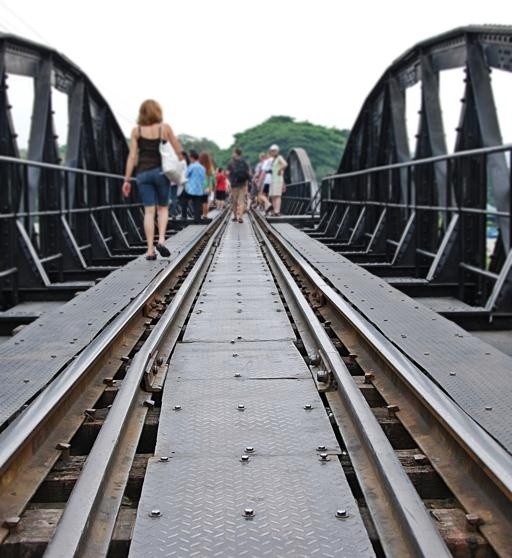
[158,125,189,185]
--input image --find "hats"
[269,144,280,152]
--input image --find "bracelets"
[124,180,130,182]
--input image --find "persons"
[168,143,288,224]
[121,98,187,261]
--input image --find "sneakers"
[145,253,157,260]
[172,205,280,225]
[155,243,170,257]
